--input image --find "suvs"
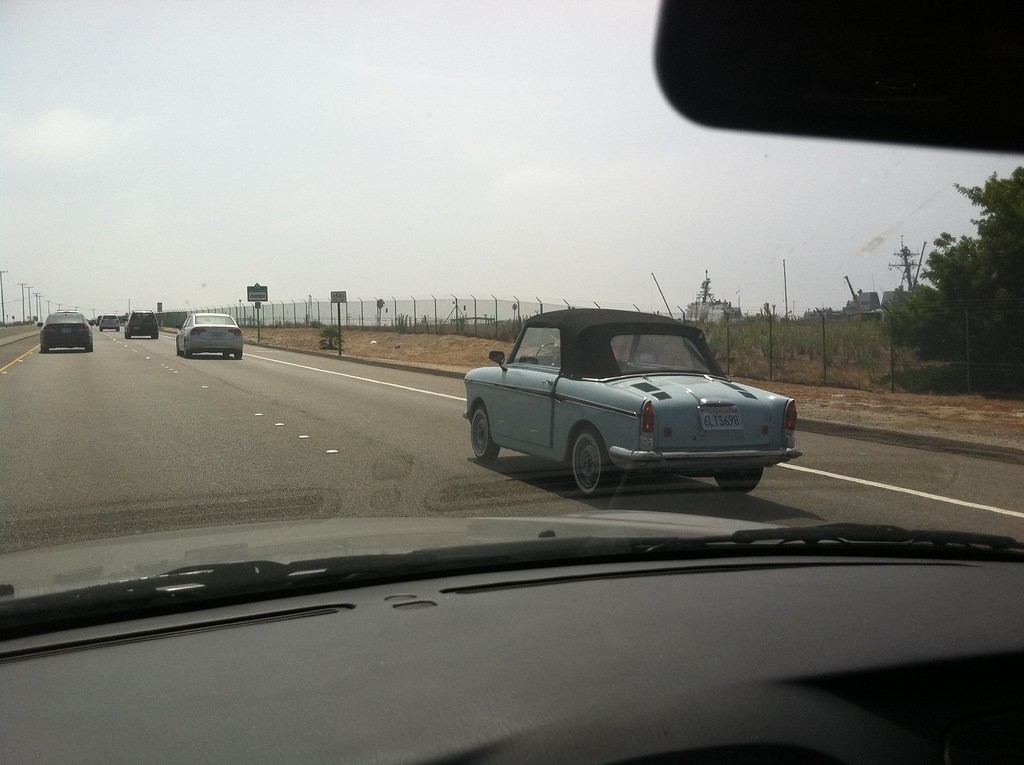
[124,310,160,339]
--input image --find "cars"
[175,312,244,360]
[36,310,94,352]
[464,308,804,498]
[95,313,120,332]
